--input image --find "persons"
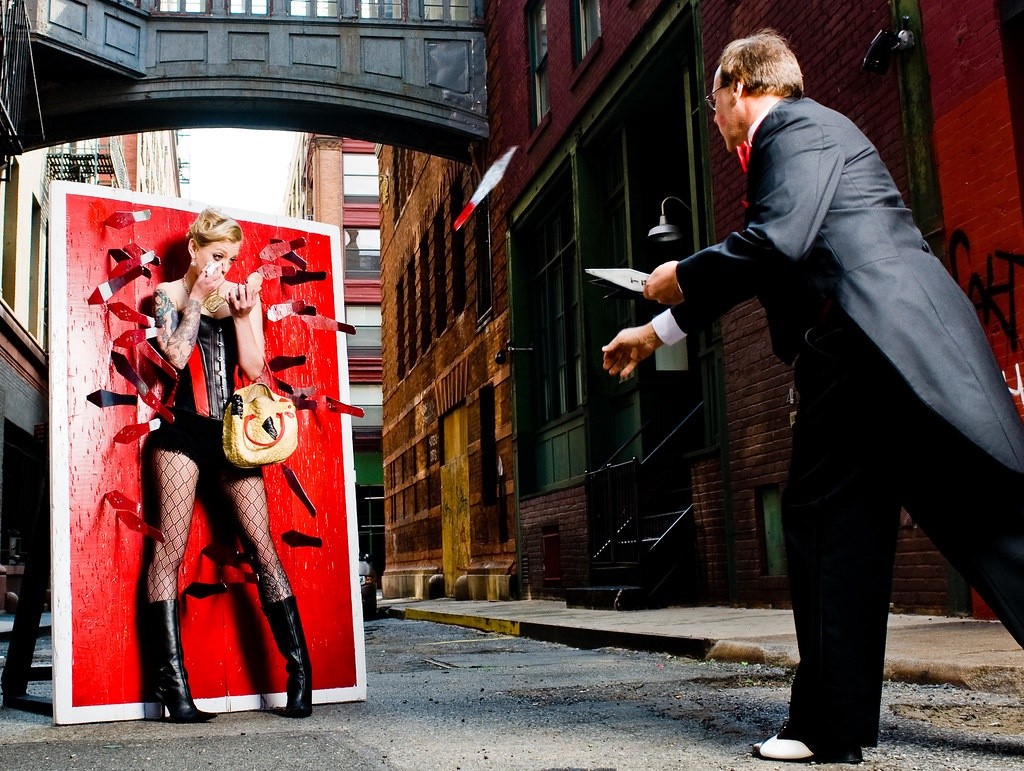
[143,206,313,721]
[603,30,1024,765]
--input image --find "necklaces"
[180,273,226,313]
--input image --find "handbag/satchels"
[220,356,299,469]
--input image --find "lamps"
[646,196,693,242]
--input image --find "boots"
[145,599,219,724]
[260,594,313,716]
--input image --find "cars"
[358,550,378,616]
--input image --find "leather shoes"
[751,720,866,765]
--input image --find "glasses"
[704,80,729,111]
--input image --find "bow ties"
[736,140,751,174]
